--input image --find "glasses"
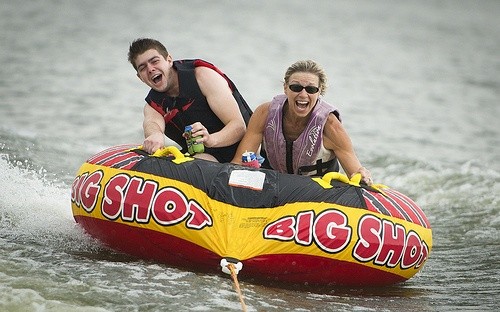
[286,83,321,95]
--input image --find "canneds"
[185,126,205,155]
[242,151,260,163]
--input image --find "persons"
[126,37,254,165]
[230,59,372,187]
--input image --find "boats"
[71,142,434,291]
